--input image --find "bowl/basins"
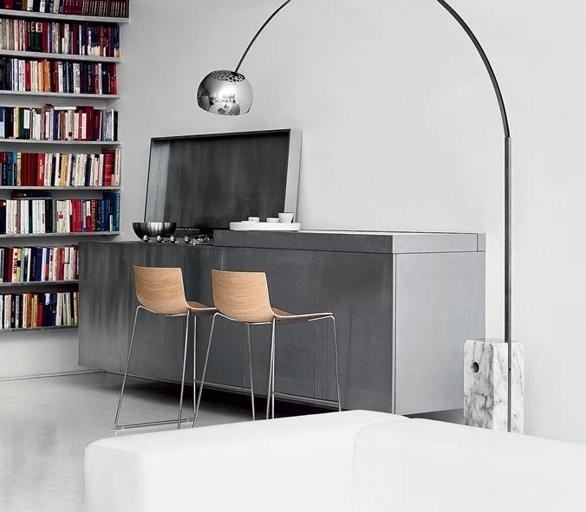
[132,222,176,241]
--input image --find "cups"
[266,218,279,223]
[277,213,294,223]
[111,174,119,187]
[249,217,260,223]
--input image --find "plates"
[230,222,301,233]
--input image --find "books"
[1,245,79,326]
[1,148,121,234]
[0,1,130,141]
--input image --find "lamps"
[199,3,513,424]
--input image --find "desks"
[81,408,582,512]
[78,233,488,414]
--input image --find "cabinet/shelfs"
[0,0,129,330]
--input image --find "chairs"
[113,265,344,428]
